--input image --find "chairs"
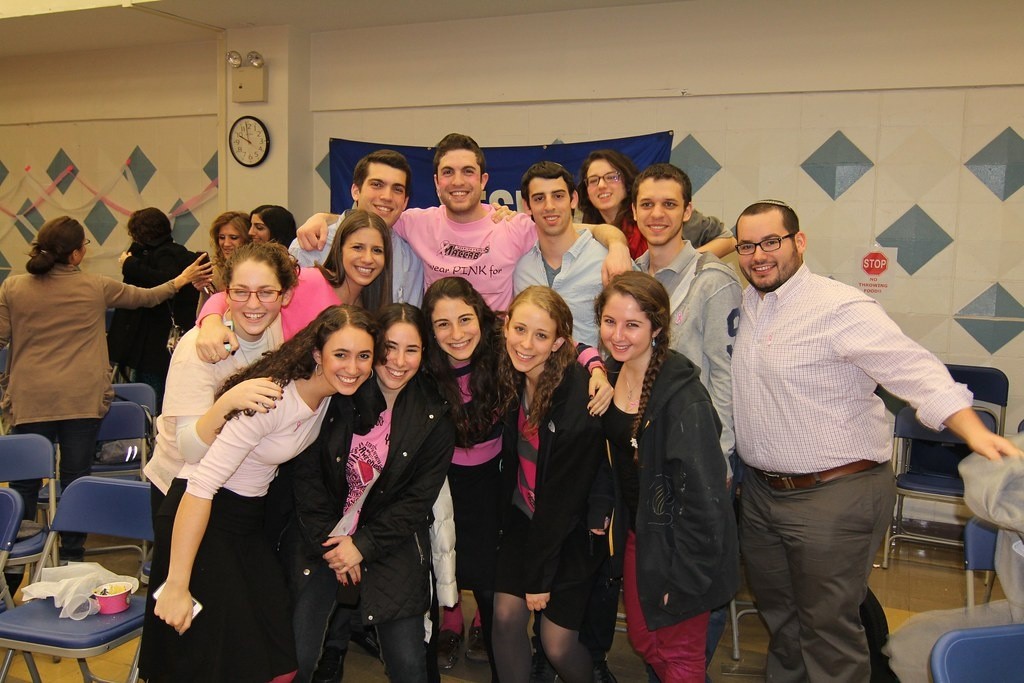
[882,365,1024,683]
[0,309,158,683]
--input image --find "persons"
[137,305,376,683]
[491,286,605,683]
[248,205,295,251]
[593,270,742,683]
[512,160,642,683]
[141,242,300,534]
[287,150,425,310]
[732,199,1023,682]
[421,277,616,670]
[119,207,201,417]
[195,209,394,364]
[490,163,744,683]
[286,302,458,683]
[0,216,214,563]
[192,211,250,320]
[574,149,738,259]
[296,132,633,323]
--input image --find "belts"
[754,460,890,491]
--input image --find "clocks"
[229,116,270,167]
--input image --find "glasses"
[582,171,623,187]
[226,288,282,303]
[77,239,90,250]
[735,233,795,256]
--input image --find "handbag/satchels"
[93,394,157,464]
[167,324,185,357]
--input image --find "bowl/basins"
[92,581,133,615]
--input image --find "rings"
[209,353,217,360]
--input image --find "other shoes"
[51,559,70,568]
[3,563,25,574]
[140,561,150,584]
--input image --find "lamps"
[226,51,268,103]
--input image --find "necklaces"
[237,325,275,367]
[623,366,644,401]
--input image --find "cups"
[66,594,101,621]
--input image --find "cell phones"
[222,320,234,351]
[126,242,145,257]
[195,251,213,276]
[153,580,204,620]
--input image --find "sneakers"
[591,652,617,683]
[528,654,558,683]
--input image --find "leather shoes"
[466,619,489,664]
[437,624,465,672]
[349,625,380,657]
[312,646,346,683]
